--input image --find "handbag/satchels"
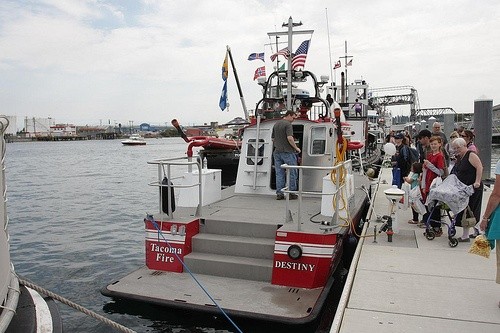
[428,174,443,190]
[461,205,476,228]
[410,198,427,216]
[392,165,401,189]
[406,182,423,201]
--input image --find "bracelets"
[482,215,490,221]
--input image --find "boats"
[96,17,388,324]
[121,133,147,144]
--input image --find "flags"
[291,40,309,71]
[218,50,230,111]
[333,60,341,69]
[347,59,352,66]
[279,63,285,71]
[248,53,264,62]
[253,66,266,80]
[270,47,290,62]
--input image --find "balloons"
[383,142,396,156]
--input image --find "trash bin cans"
[207,150,240,186]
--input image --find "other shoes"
[289,193,298,200]
[469,234,477,238]
[423,227,443,237]
[457,237,470,242]
[276,194,284,200]
[417,221,427,228]
[408,219,418,224]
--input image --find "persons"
[326,94,333,106]
[351,99,363,120]
[385,122,483,243]
[423,136,446,237]
[271,110,301,200]
[479,158,500,285]
[448,137,484,243]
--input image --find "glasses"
[450,136,456,138]
[459,133,467,137]
